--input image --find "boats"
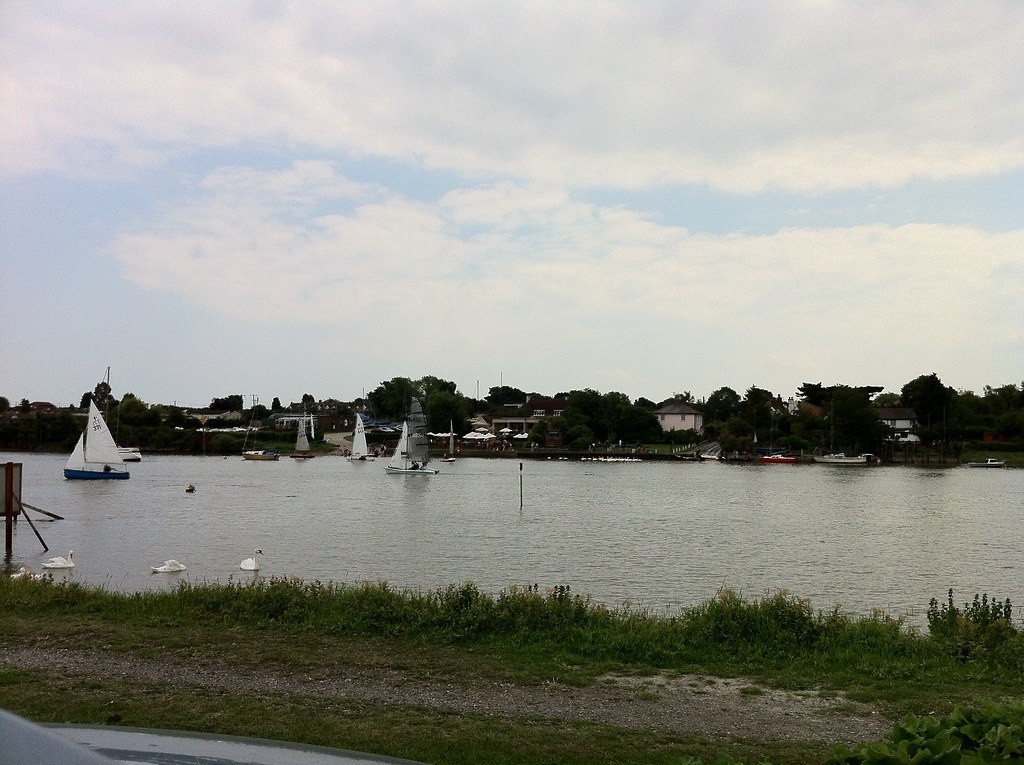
[114,445,142,462]
[969,458,1006,468]
[242,450,279,461]
[812,453,867,463]
[759,454,800,464]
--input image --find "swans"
[150,560,187,573]
[41,550,74,568]
[239,549,264,571]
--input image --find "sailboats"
[440,419,456,462]
[63,398,130,479]
[385,395,440,478]
[346,413,375,462]
[289,418,316,458]
